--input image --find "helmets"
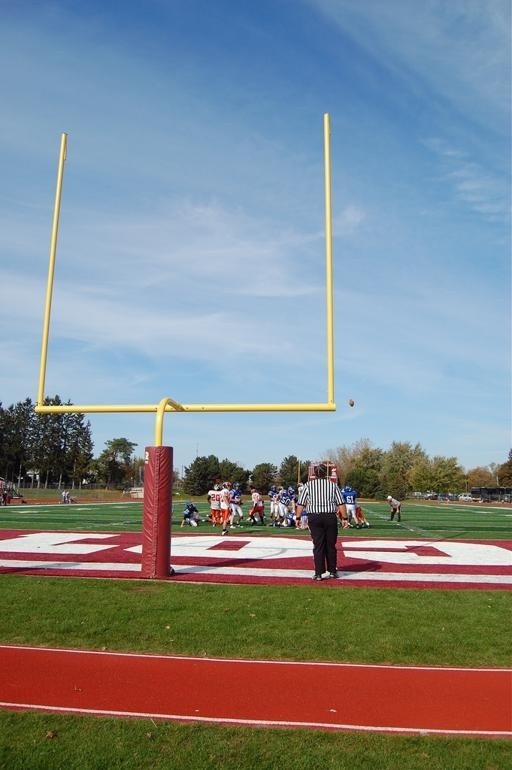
[314,464,327,478]
[271,486,276,492]
[233,482,240,488]
[250,489,256,493]
[223,482,231,487]
[214,483,220,491]
[341,485,352,493]
[186,502,193,508]
[278,486,283,491]
[288,486,295,495]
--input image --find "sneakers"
[330,572,339,578]
[312,574,322,581]
[201,514,241,535]
[347,521,370,529]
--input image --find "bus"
[471,487,512,503]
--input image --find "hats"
[387,495,392,500]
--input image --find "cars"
[406,492,471,502]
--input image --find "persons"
[66,490,70,503]
[294,464,349,581]
[181,478,370,537]
[61,490,66,503]
[2,491,8,506]
[386,496,401,522]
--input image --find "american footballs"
[349,400,354,406]
